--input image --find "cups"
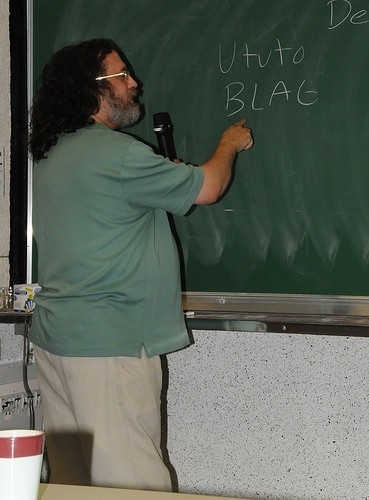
[0,429,44,500]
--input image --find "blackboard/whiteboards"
[8,1,369,316]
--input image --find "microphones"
[153,112,177,162]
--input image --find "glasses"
[96,69,130,81]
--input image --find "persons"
[29,38,254,493]
